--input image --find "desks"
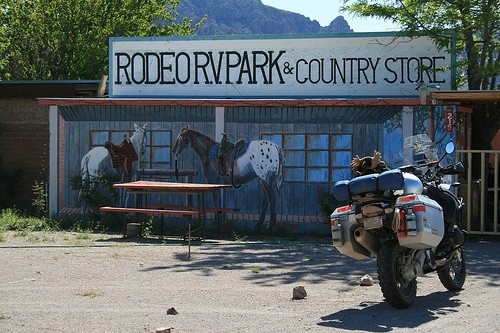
[112,179,233,241]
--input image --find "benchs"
[144,203,241,239]
[100,205,203,245]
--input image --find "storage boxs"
[330,203,372,261]
[394,193,445,250]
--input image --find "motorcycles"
[329,134,470,309]
